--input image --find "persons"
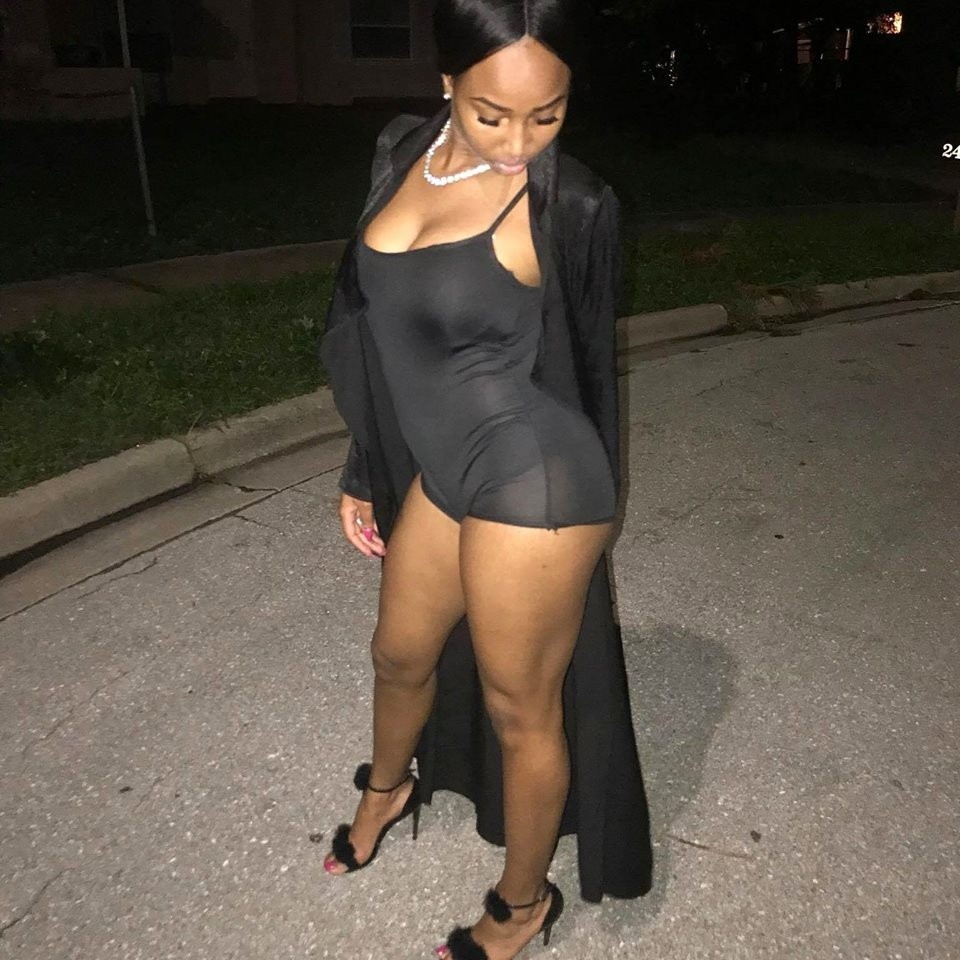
[321,0,654,960]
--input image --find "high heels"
[447,879,564,960]
[333,774,421,873]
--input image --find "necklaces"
[422,116,494,186]
